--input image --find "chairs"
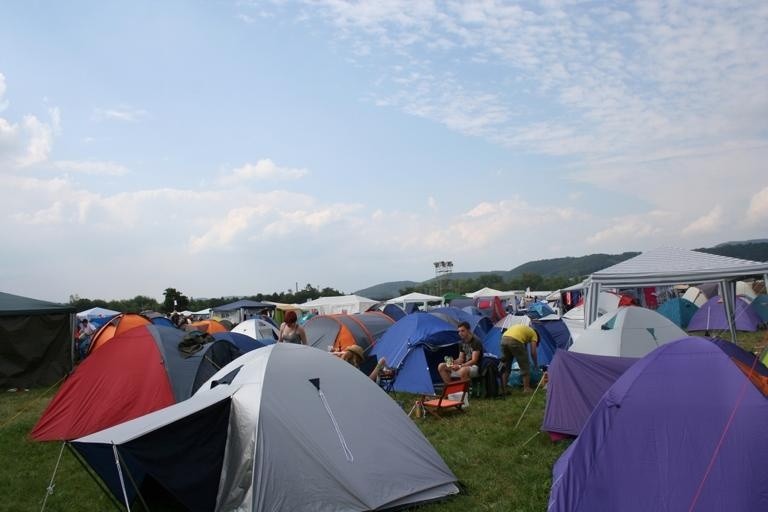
[418,378,471,420]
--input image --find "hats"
[347,344,365,360]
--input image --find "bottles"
[416,401,422,419]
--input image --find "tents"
[544,334,768,512]
[66,342,460,512]
[537,304,692,443]
[1,285,768,405]
[26,321,241,445]
